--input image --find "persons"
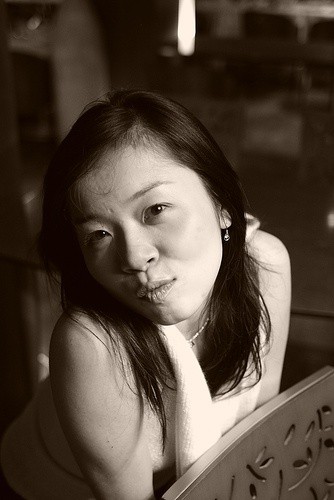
[0,89,292,500]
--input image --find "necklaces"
[186,310,212,351]
